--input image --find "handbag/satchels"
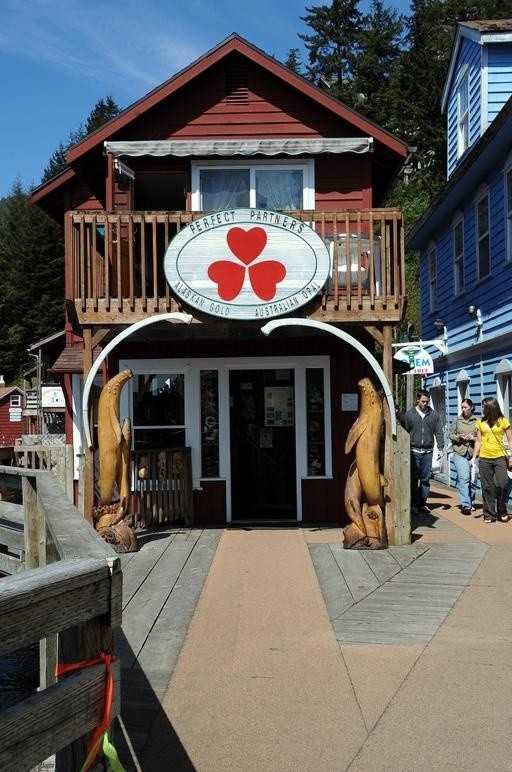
[504,455,512,471]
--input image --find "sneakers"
[461,507,471,514]
[419,505,431,513]
[471,506,475,511]
[411,505,418,514]
[484,518,491,523]
[500,515,509,522]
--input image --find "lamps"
[467,303,477,316]
[433,318,446,331]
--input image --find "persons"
[394,404,403,420]
[398,389,445,515]
[448,398,481,514]
[467,397,511,523]
